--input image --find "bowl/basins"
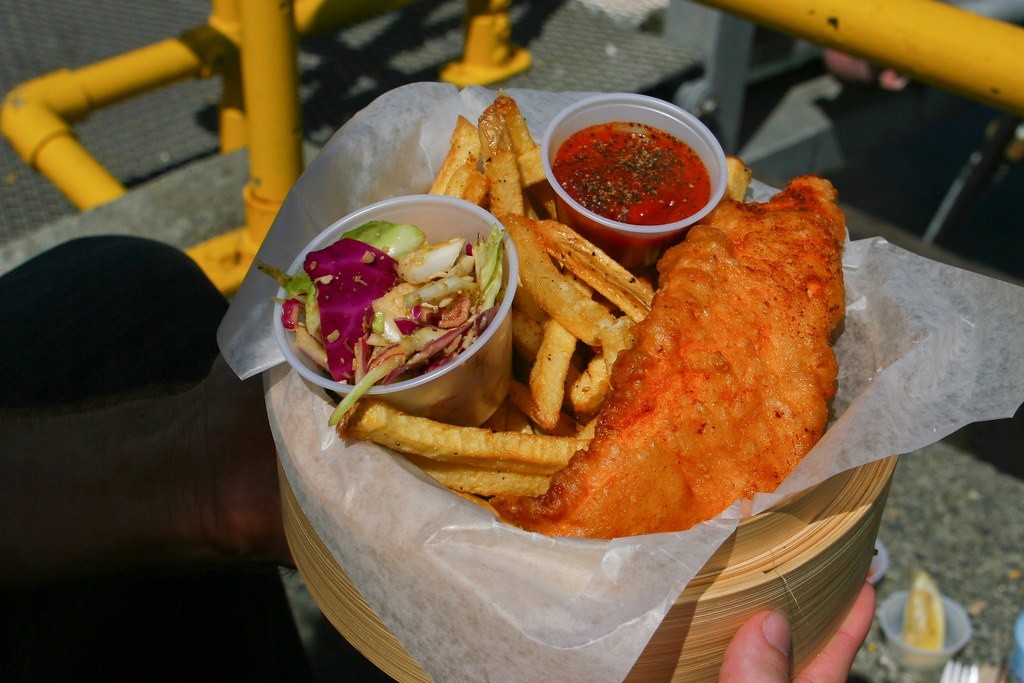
[877,594,971,671]
[865,541,890,585]
[273,194,518,427]
[542,94,729,269]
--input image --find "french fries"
[330,92,754,505]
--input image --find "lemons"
[901,569,944,653]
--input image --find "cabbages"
[255,222,505,426]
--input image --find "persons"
[0,237,876,683]
